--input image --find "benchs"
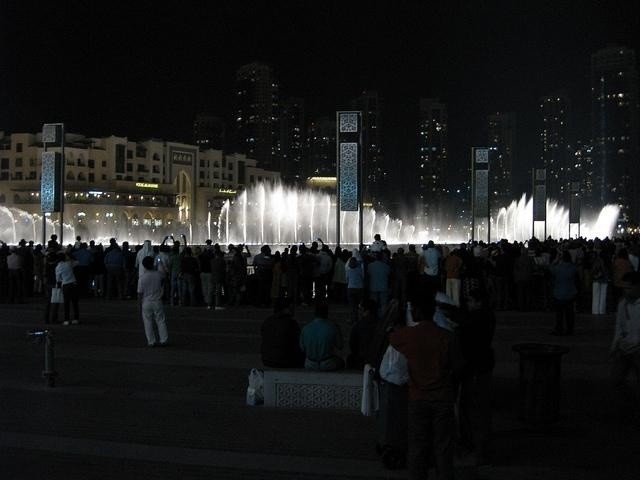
[263,369,378,415]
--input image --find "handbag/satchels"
[51,282,65,304]
[379,345,410,387]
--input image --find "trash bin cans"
[512,343,571,426]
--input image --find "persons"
[249,235,640,480]
[1,236,252,348]
